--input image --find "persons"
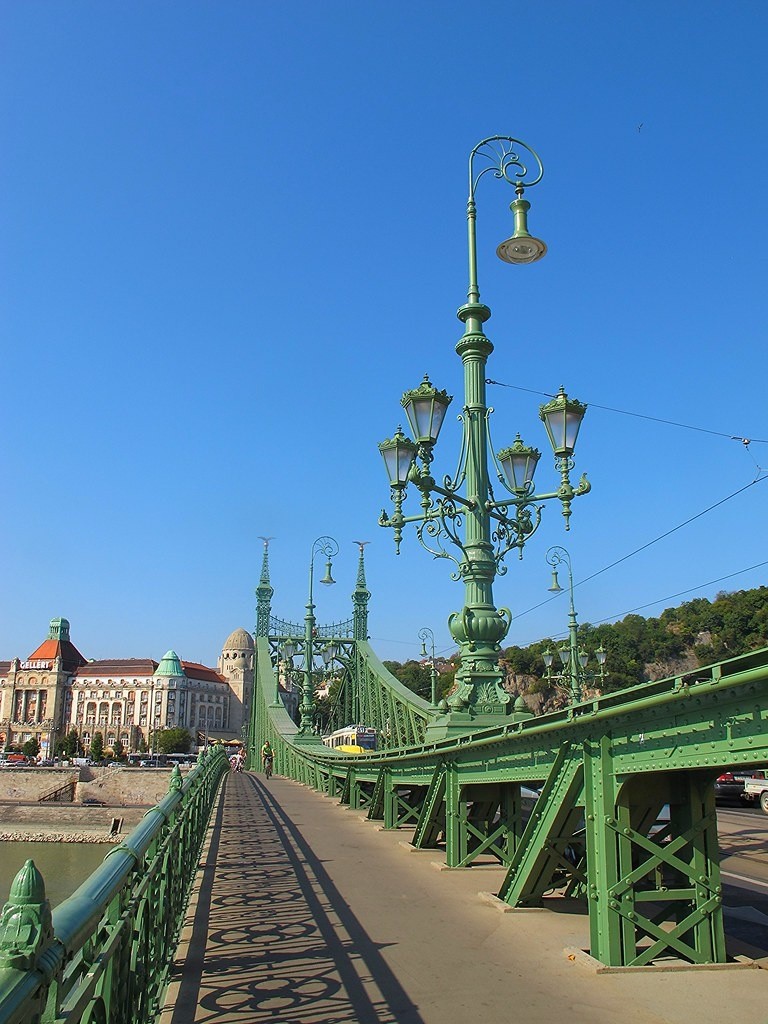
[238,749,247,763]
[261,740,277,774]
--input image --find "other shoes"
[232,764,273,777]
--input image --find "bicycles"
[262,755,275,779]
[229,752,246,774]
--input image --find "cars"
[0,758,192,768]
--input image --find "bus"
[128,753,199,766]
[321,723,379,759]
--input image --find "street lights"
[290,534,339,736]
[417,624,441,712]
[376,129,594,719]
[542,543,609,716]
